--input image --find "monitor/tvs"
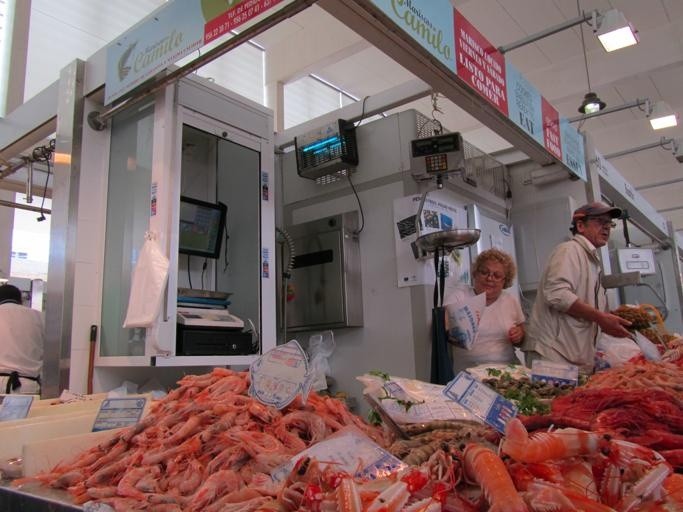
[179,195,227,260]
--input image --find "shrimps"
[457,351,681,512]
[36,364,456,512]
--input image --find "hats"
[573,201,622,221]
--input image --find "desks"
[0,468,95,512]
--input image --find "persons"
[443,248,526,377]
[0,285,45,394]
[520,202,634,375]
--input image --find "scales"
[409,132,482,253]
[176,287,245,330]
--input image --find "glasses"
[475,270,506,282]
[587,218,617,227]
